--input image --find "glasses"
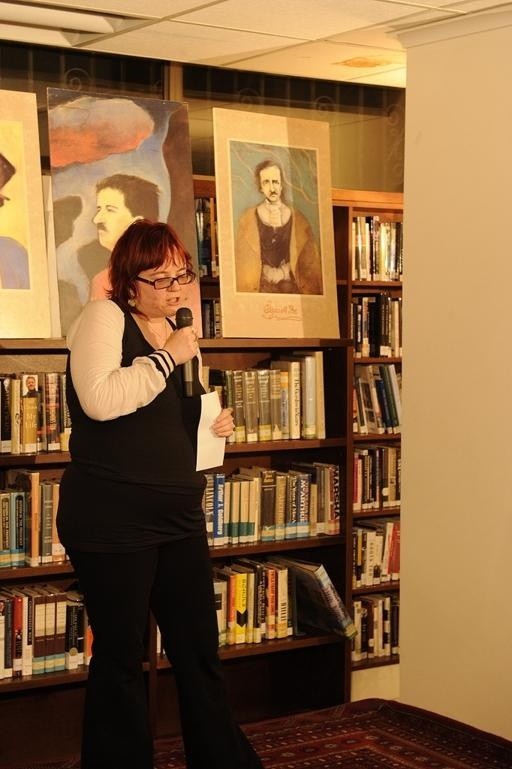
[134,269,196,290]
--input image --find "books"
[202,464,342,546]
[350,215,403,359]
[0,470,69,568]
[0,585,167,678]
[353,364,400,662]
[213,556,359,648]
[0,372,73,454]
[202,350,325,442]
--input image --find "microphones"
[175,307,195,397]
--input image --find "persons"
[237,161,320,293]
[53,220,265,769]
[66,174,160,348]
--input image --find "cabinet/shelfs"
[1,174,352,749]
[345,189,408,670]
